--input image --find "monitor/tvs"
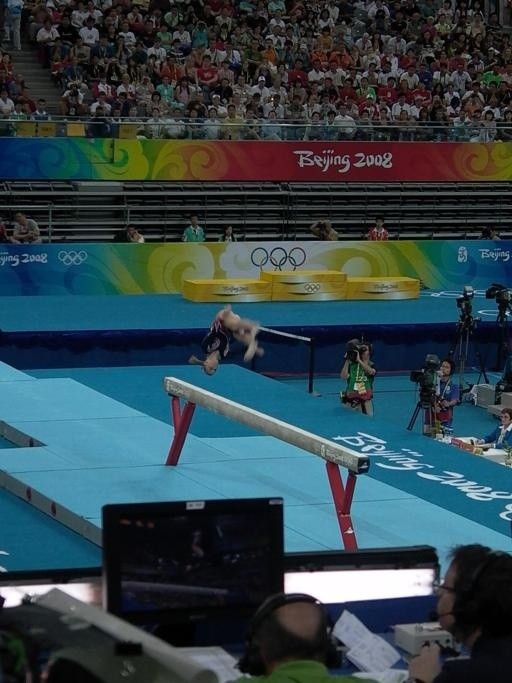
[101,496,287,650]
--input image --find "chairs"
[0,0,512,142]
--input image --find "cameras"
[320,223,326,231]
[70,89,79,97]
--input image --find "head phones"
[244,593,343,677]
[455,550,508,607]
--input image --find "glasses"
[432,578,455,595]
[500,415,509,421]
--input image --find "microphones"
[430,608,465,622]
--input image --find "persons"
[224,593,378,683]
[188,304,265,376]
[0,212,42,244]
[369,217,388,240]
[218,224,237,242]
[408,544,512,683]
[475,408,512,449]
[340,342,377,416]
[181,214,206,242]
[424,358,459,427]
[0,0,512,143]
[310,219,338,241]
[478,226,502,240]
[127,224,144,244]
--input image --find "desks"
[183,626,474,683]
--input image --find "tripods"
[447,321,489,405]
[407,401,445,439]
[483,318,509,372]
